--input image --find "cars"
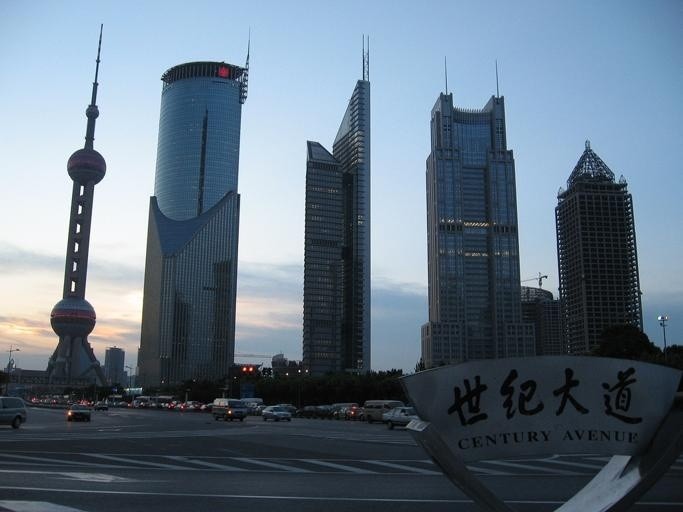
[67,403,92,422]
[382,406,417,430]
[0,396,28,430]
